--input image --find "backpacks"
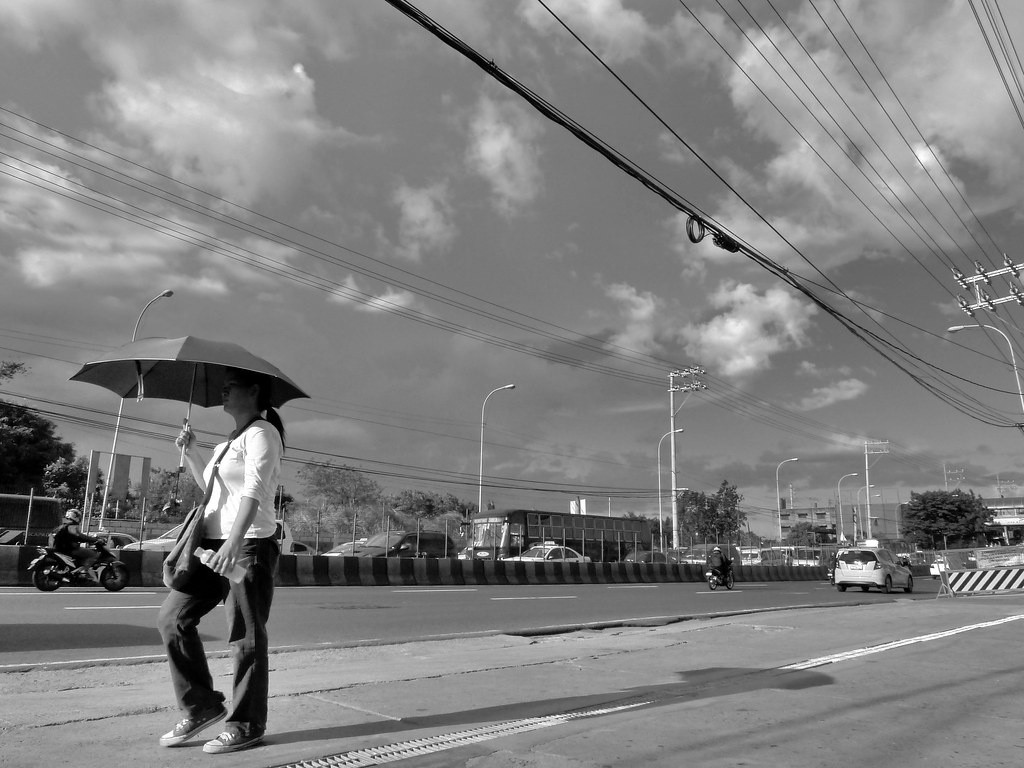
[48,524,68,549]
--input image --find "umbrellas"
[69,335,312,473]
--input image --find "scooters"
[828,566,835,586]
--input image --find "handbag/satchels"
[161,505,204,590]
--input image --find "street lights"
[837,472,858,546]
[895,502,908,545]
[947,324,1024,415]
[857,485,876,540]
[776,458,800,541]
[98,289,175,535]
[865,495,881,540]
[478,384,516,513]
[657,428,685,554]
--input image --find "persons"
[903,556,911,568]
[156,374,285,754]
[58,509,106,580]
[709,546,733,585]
[829,553,835,570]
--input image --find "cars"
[930,553,977,579]
[497,540,592,562]
[294,537,370,557]
[834,546,914,594]
[623,542,840,567]
[78,531,139,550]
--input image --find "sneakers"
[204,730,265,752]
[159,699,227,746]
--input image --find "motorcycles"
[27,533,131,592]
[705,556,735,590]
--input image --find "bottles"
[193,547,247,583]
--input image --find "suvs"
[338,529,459,559]
[122,519,295,556]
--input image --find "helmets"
[713,547,721,552]
[65,509,82,525]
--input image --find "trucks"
[457,507,654,562]
